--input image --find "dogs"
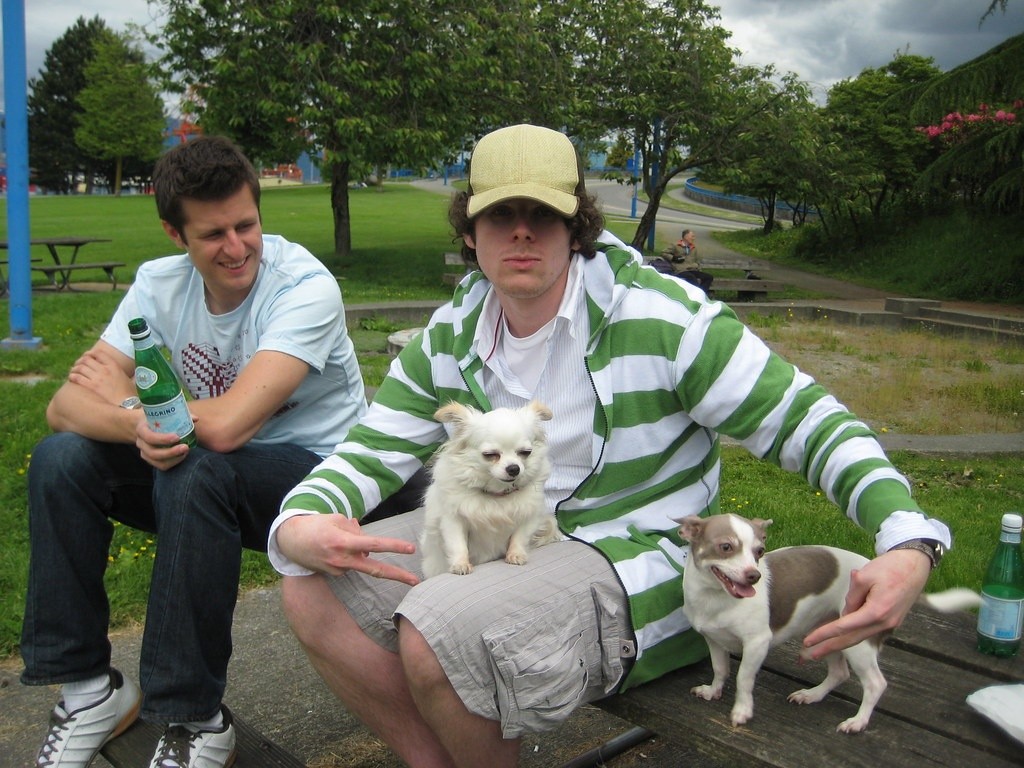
[666,513,992,735]
[417,399,559,579]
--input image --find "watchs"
[121,396,141,409]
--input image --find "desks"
[0,237,111,291]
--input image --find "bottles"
[977,514,1024,657]
[128,318,199,454]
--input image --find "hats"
[466,123,580,217]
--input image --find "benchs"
[30,262,126,291]
[442,253,785,300]
[0,258,43,295]
[588,598,1024,768]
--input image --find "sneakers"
[35,667,142,768]
[149,703,238,768]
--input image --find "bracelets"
[895,542,944,569]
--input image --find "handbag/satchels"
[650,258,672,273]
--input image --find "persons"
[661,230,713,292]
[265,124,952,768]
[18,136,369,768]
